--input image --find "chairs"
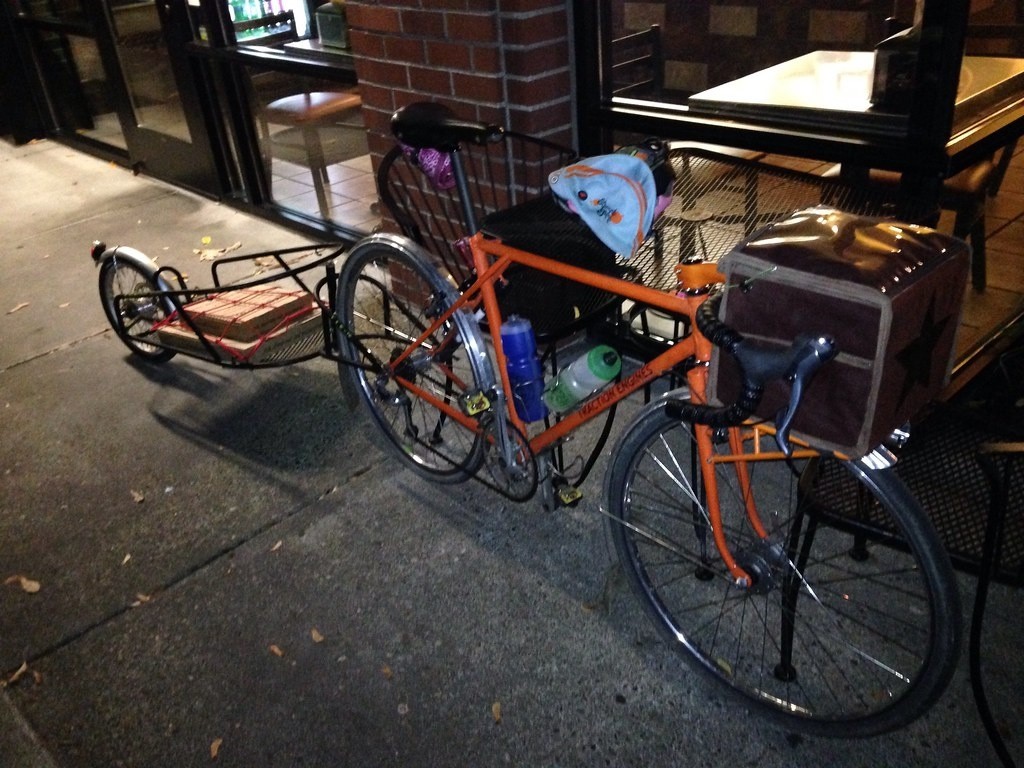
[774,402,1024,768]
[819,16,1015,290]
[611,24,678,194]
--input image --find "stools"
[259,86,363,221]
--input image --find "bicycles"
[335,100,962,742]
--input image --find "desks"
[693,49,1024,296]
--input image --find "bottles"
[542,345,621,412]
[227,0,290,40]
[500,315,550,423]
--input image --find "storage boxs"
[704,201,965,460]
